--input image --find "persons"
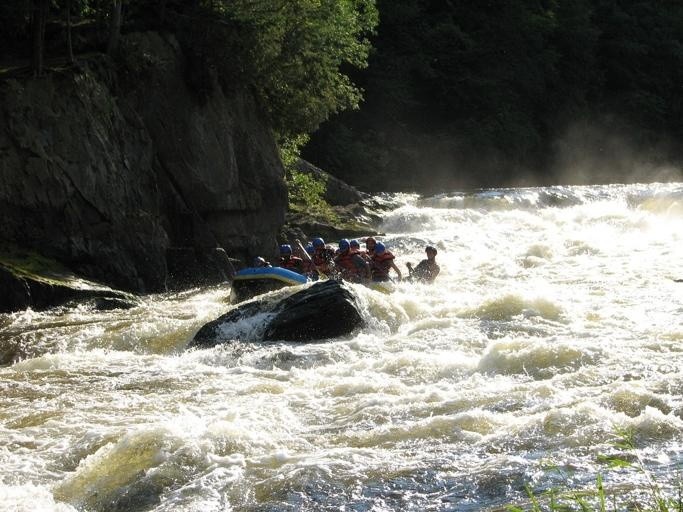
[254,256,271,267]
[279,237,402,287]
[394,246,440,286]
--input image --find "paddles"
[336,267,390,297]
[293,239,328,280]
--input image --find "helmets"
[326,245,334,251]
[339,240,349,250]
[306,238,324,253]
[366,238,375,241]
[375,243,385,252]
[426,246,437,255]
[255,257,265,267]
[280,244,292,253]
[350,240,359,246]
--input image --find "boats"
[232,267,397,299]
